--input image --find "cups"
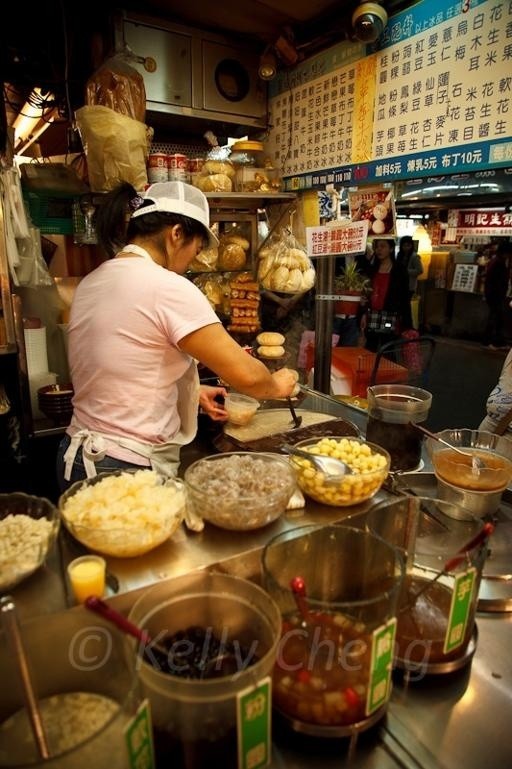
[367,386,430,471]
[126,570,282,768]
[2,614,145,767]
[69,556,105,602]
[262,523,404,726]
[366,498,487,665]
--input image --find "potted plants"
[333,257,373,315]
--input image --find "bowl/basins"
[0,492,57,591]
[188,452,295,531]
[293,437,390,510]
[38,384,75,423]
[224,390,260,425]
[429,427,511,486]
[270,391,306,407]
[434,473,506,521]
[61,469,187,555]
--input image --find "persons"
[53,180,299,491]
[484,240,512,345]
[477,347,512,460]
[332,235,423,351]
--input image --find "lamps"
[258,30,305,81]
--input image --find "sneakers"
[480,344,511,351]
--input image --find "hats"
[131,181,220,250]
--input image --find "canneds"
[149,152,167,182]
[171,153,203,184]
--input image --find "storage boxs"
[305,345,409,398]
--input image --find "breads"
[218,236,252,269]
[257,331,284,345]
[259,245,315,293]
[227,273,260,334]
[191,275,229,312]
[257,346,286,357]
[193,160,236,190]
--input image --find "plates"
[391,460,426,476]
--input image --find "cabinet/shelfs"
[86,193,297,345]
[98,10,267,137]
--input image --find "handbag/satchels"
[401,329,422,375]
[365,310,397,334]
